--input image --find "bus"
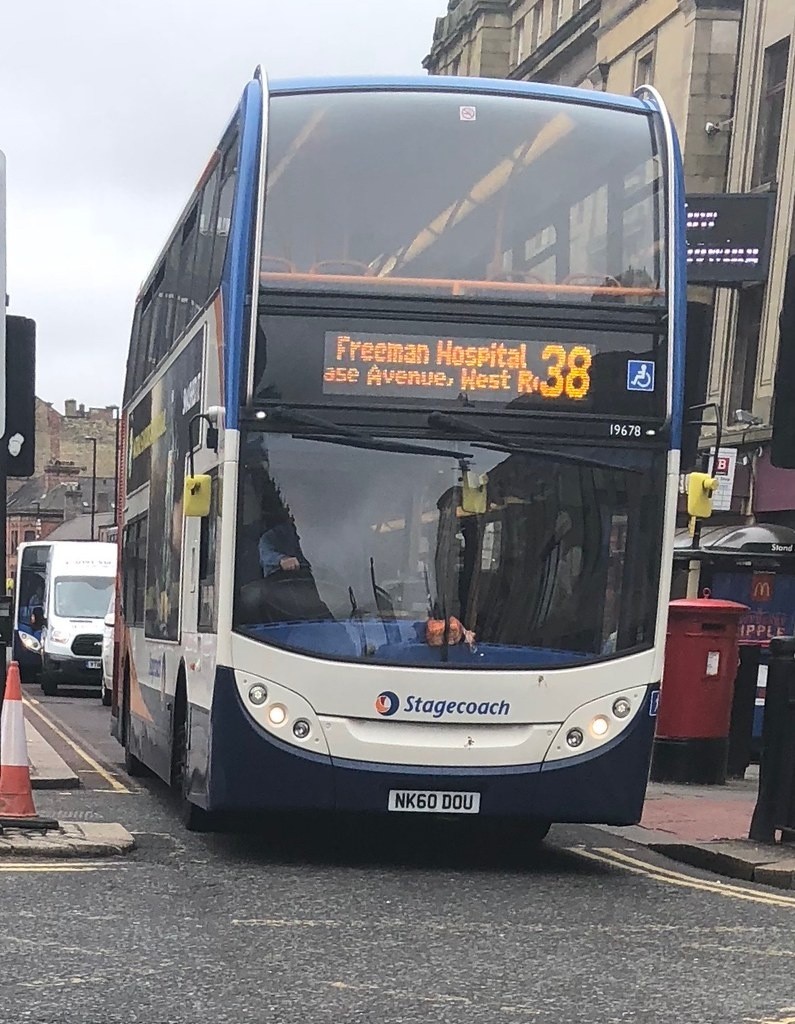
[10,539,71,685]
[105,60,727,853]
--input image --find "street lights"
[85,435,98,541]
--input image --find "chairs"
[257,256,626,303]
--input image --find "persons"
[259,478,340,578]
[29,585,43,606]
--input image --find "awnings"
[672,523,795,563]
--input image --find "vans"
[100,586,120,708]
[30,540,120,692]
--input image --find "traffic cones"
[0,660,59,830]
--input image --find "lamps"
[735,409,764,427]
